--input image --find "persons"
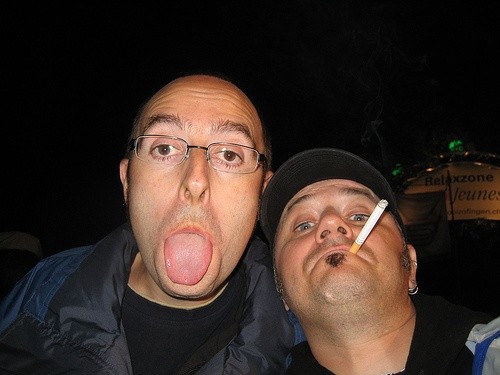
[0,74,307,375]
[260,148,500,375]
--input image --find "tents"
[386,160,500,227]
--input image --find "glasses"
[129,134,268,174]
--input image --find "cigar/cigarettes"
[350,200,389,255]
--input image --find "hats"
[260,148,407,243]
